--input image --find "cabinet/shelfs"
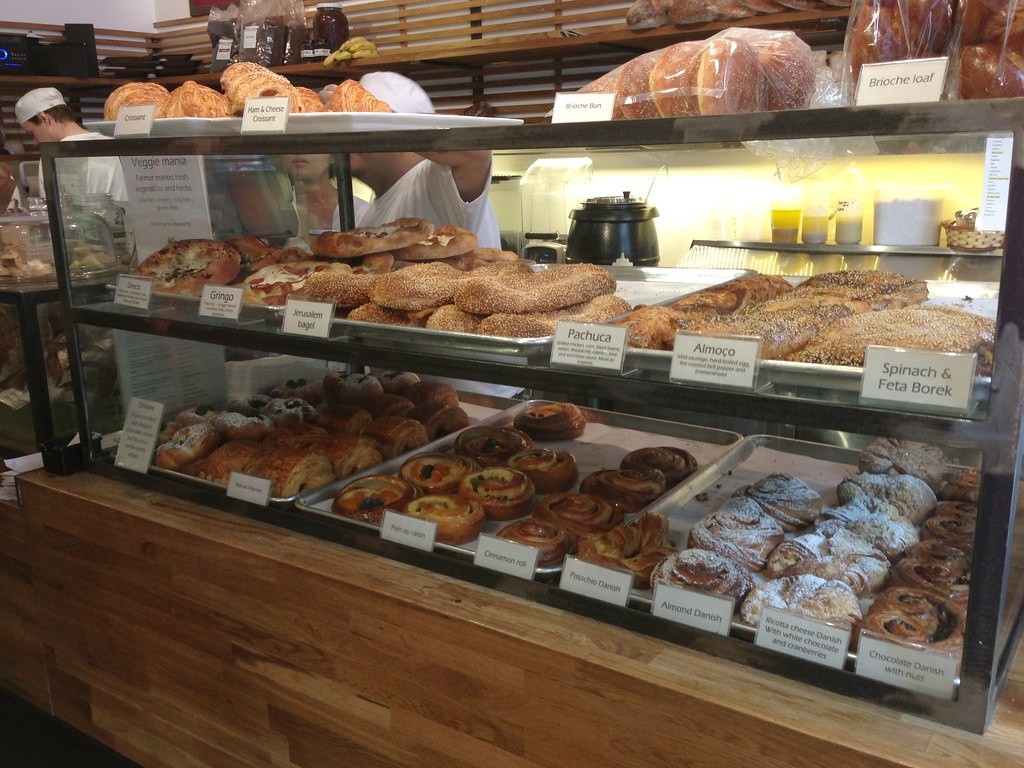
[40,97,1024,736]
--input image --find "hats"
[359,71,436,114]
[15,87,67,125]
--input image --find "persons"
[259,153,370,254]
[15,85,128,218]
[318,71,501,251]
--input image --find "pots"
[565,191,659,266]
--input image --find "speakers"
[39,24,100,79]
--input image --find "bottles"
[313,3,348,61]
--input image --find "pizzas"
[132,217,517,309]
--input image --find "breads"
[300,247,994,381]
[575,0,1024,116]
[0,236,118,443]
[98,62,390,124]
[149,369,982,660]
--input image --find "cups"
[802,204,827,244]
[835,204,862,243]
[771,205,800,245]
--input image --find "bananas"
[323,37,378,65]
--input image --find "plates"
[83,110,522,137]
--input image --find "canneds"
[297,3,349,63]
